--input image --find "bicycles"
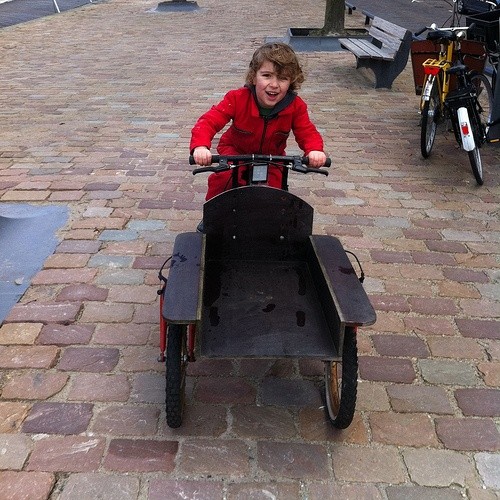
[409,0,497,158]
[414,22,497,184]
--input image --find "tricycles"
[156,153,377,428]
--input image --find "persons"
[189,41,327,235]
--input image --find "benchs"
[338,15,413,90]
